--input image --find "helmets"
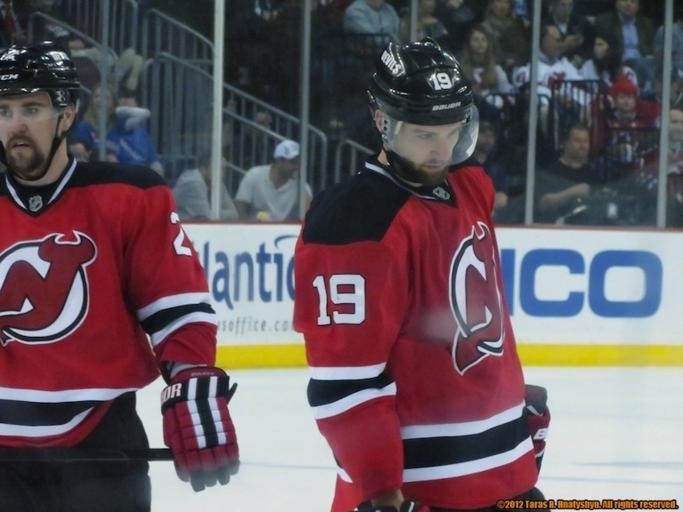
[0,38,81,108]
[364,36,480,167]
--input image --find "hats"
[274,139,299,160]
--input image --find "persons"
[0,0,682,224]
[0,41,239,512]
[293,35,550,512]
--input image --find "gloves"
[524,384,550,470]
[161,366,240,491]
